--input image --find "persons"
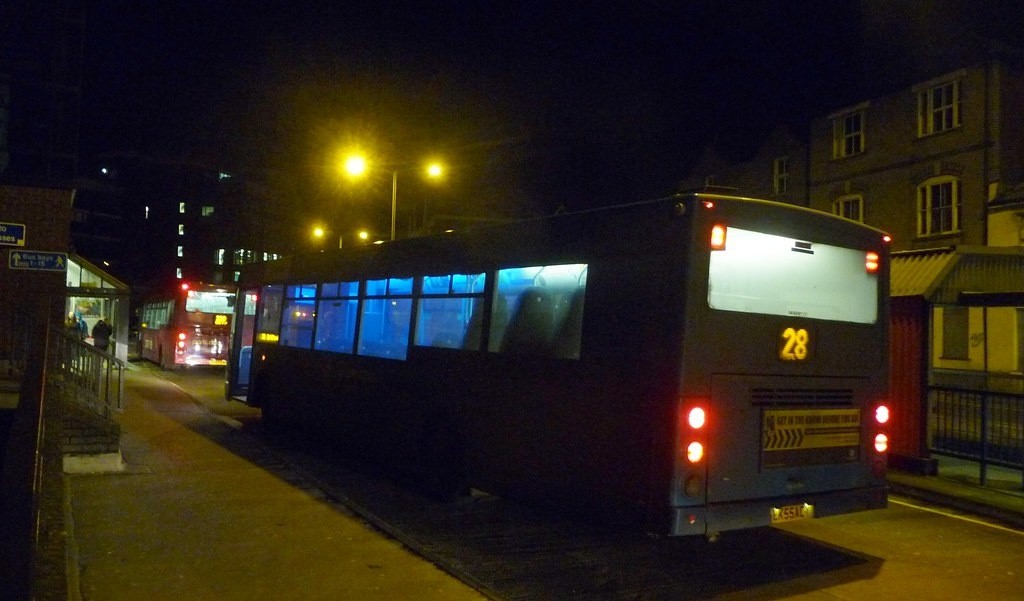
[65,310,112,366]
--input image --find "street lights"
[345,155,448,241]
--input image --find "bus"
[224,193,895,542]
[134,281,237,373]
